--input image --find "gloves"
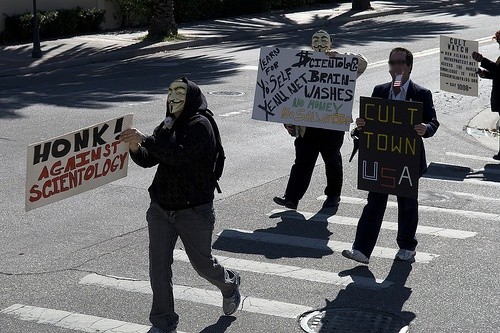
[472,52,482,62]
[476,68,484,76]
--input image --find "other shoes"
[493,153,500,160]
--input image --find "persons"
[119,75,241,333]
[472,31,500,160]
[272,30,367,209]
[342,48,440,265]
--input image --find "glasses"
[388,59,406,66]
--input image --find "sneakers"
[147,325,179,333]
[394,249,415,260]
[324,197,340,206]
[222,275,241,314]
[273,195,298,210]
[342,249,368,263]
